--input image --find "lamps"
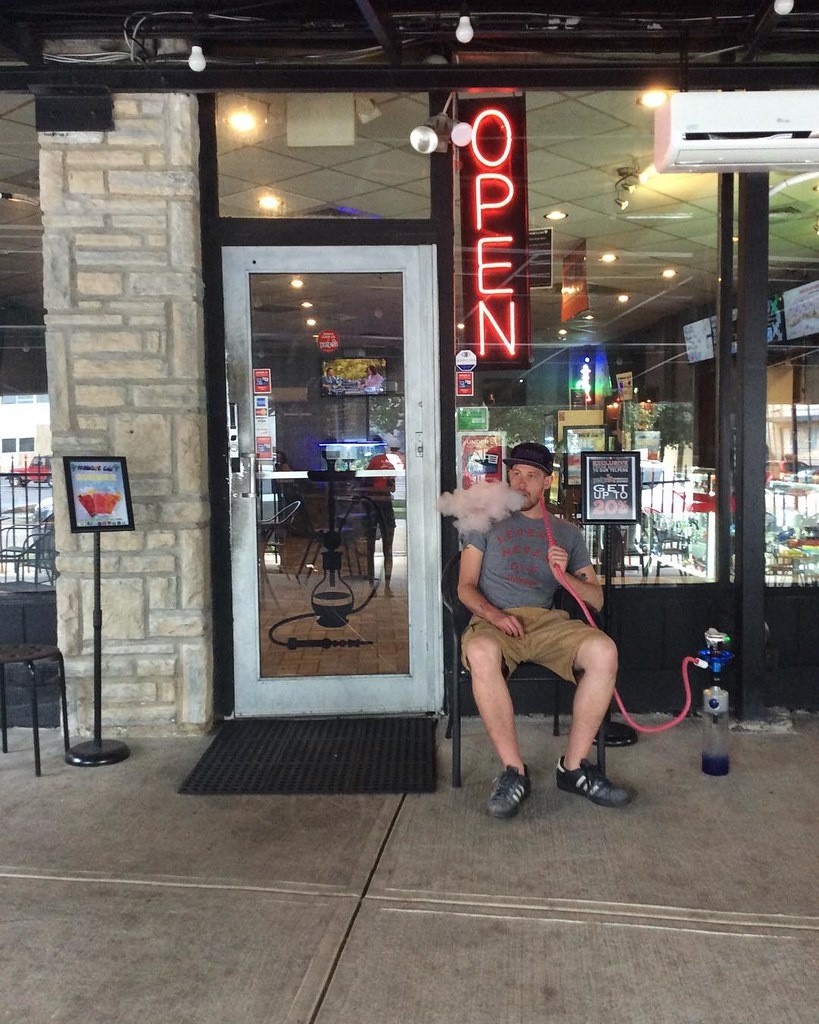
[407,92,473,154]
[613,170,647,210]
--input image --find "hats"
[504,442,553,475]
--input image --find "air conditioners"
[655,87,819,174]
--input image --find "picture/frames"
[580,451,642,527]
[62,456,135,533]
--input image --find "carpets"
[177,716,438,795]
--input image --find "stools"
[295,511,379,580]
[0,641,70,776]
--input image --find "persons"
[321,367,341,395]
[362,435,396,600]
[454,443,632,820]
[275,451,292,471]
[357,365,386,394]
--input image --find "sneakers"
[485,764,531,817]
[556,756,631,808]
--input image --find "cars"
[765,457,819,491]
[4,455,55,487]
[639,457,691,516]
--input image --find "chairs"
[440,551,607,789]
[255,500,302,609]
